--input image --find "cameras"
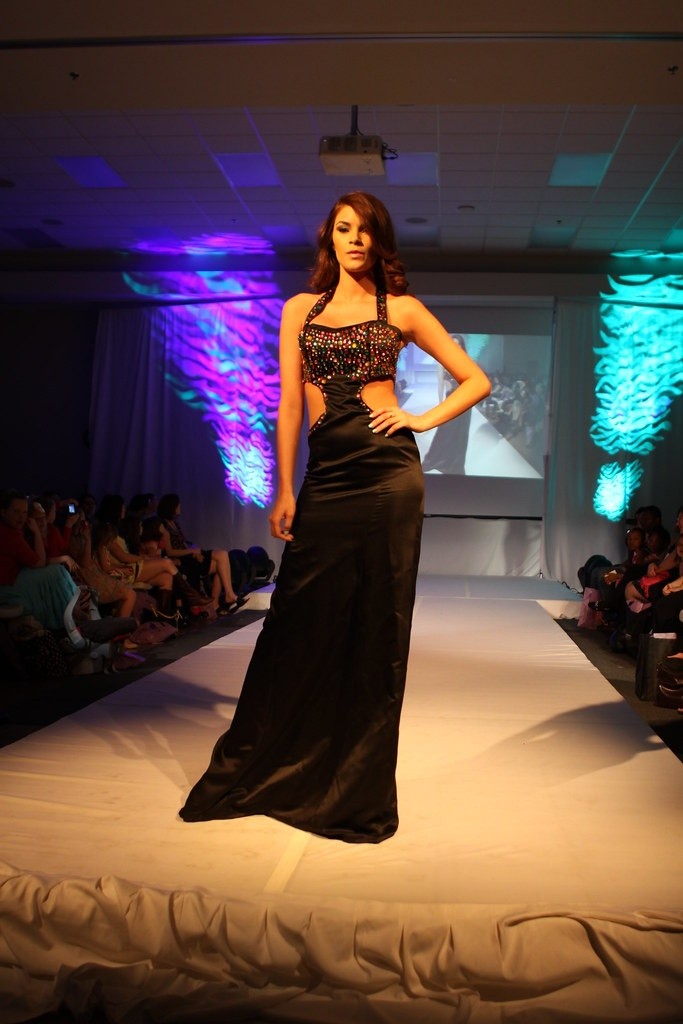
[67,503,76,515]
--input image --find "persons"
[482,371,546,445]
[577,506,683,715]
[0,490,249,676]
[179,191,492,843]
[422,335,473,475]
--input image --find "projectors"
[318,134,386,176]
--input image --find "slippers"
[228,596,251,614]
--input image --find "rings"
[390,412,392,417]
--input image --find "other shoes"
[188,590,214,608]
[587,601,615,613]
[659,683,683,699]
